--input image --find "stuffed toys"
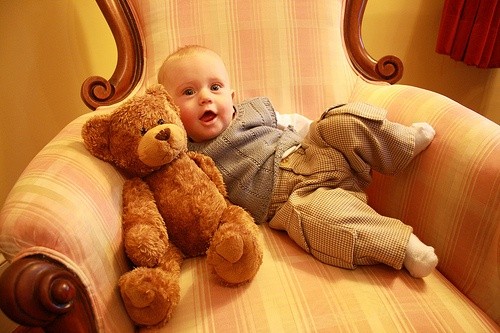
[81,83,264,329]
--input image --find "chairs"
[0,0,500,333]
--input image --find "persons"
[158,46,439,278]
